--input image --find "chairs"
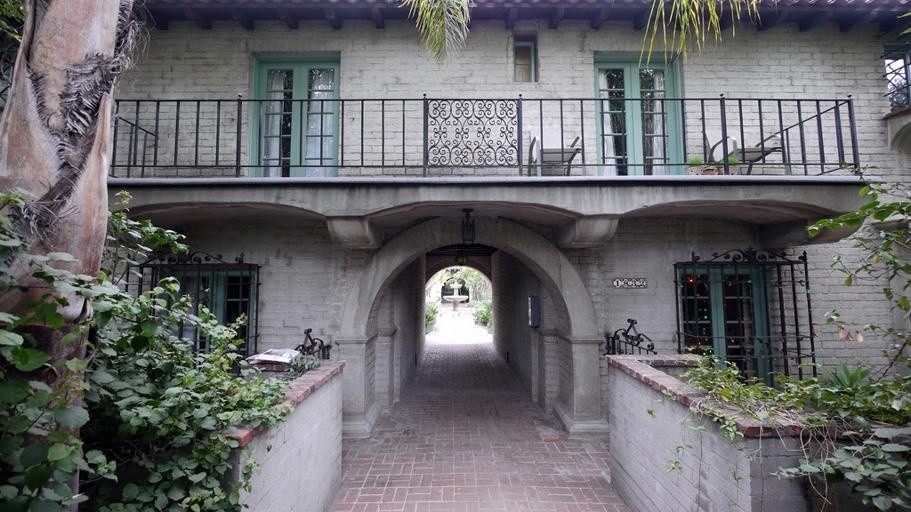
[704,129,783,176]
[527,124,582,176]
